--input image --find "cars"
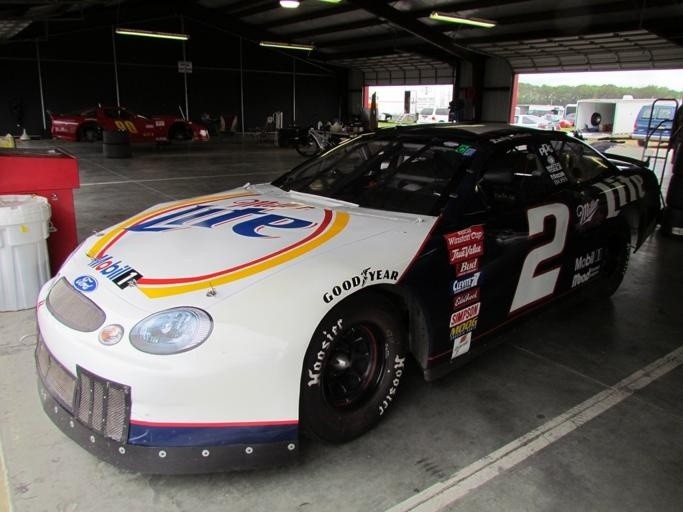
[379,103,578,134]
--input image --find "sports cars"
[43,106,210,143]
[27,123,662,474]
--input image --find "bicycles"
[293,125,354,158]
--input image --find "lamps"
[428,1,498,29]
[113,1,191,42]
[258,28,316,52]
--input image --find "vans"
[630,104,677,148]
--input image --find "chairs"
[385,146,536,209]
